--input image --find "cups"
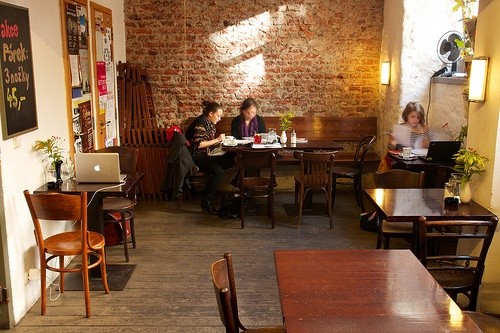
[403,146,412,160]
[443,173,462,200]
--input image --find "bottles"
[254,128,296,144]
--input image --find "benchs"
[117,60,170,205]
[208,117,382,185]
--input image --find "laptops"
[74,153,127,183]
[417,141,461,162]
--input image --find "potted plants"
[278,112,295,144]
[33,135,67,184]
[449,148,489,203]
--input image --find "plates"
[221,137,254,146]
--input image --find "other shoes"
[200,200,217,215]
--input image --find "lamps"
[380,60,392,86]
[469,57,489,102]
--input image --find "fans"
[437,29,466,77]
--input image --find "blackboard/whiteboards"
[0,1,38,140]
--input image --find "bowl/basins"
[226,136,234,141]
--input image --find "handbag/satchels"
[377,155,392,173]
[218,191,249,220]
[360,210,380,232]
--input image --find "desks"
[363,188,497,260]
[33,170,145,290]
[272,249,483,333]
[220,139,343,217]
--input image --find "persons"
[230,98,265,212]
[390,101,429,150]
[186,101,237,212]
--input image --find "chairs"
[168,126,206,212]
[88,145,140,262]
[24,189,110,318]
[211,252,288,333]
[376,169,425,256]
[293,149,339,230]
[233,148,278,231]
[414,211,499,313]
[326,135,376,210]
